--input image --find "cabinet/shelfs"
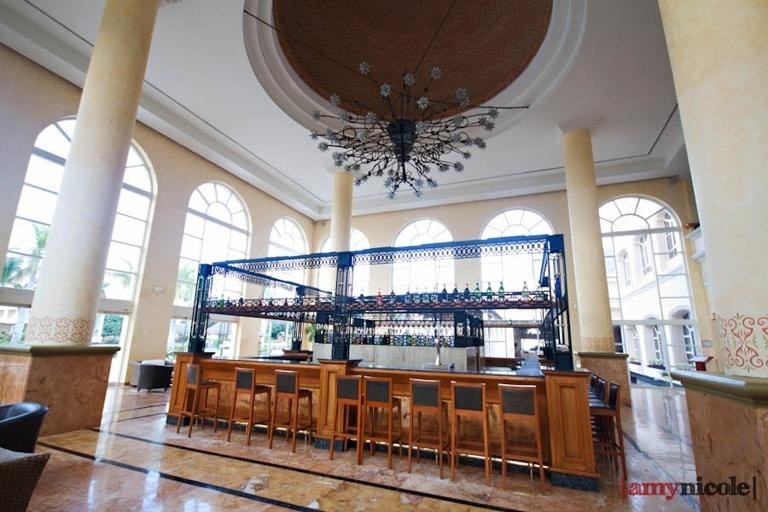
[188,233,573,370]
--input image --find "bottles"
[206,281,549,346]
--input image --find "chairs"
[450,380,496,486]
[0,402,50,453]
[498,383,545,491]
[406,377,450,479]
[137,363,174,393]
[269,371,313,454]
[363,377,403,469]
[227,367,271,447]
[130,359,174,389]
[329,374,372,465]
[0,446,49,512]
[176,363,220,437]
[589,370,628,479]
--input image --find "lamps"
[309,58,500,201]
